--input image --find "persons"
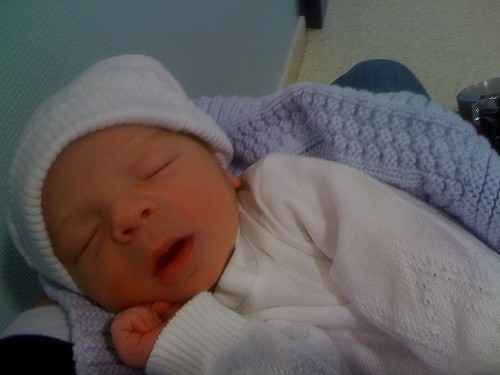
[0,57,431,375]
[5,55,500,375]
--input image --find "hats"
[10,51,236,293]
